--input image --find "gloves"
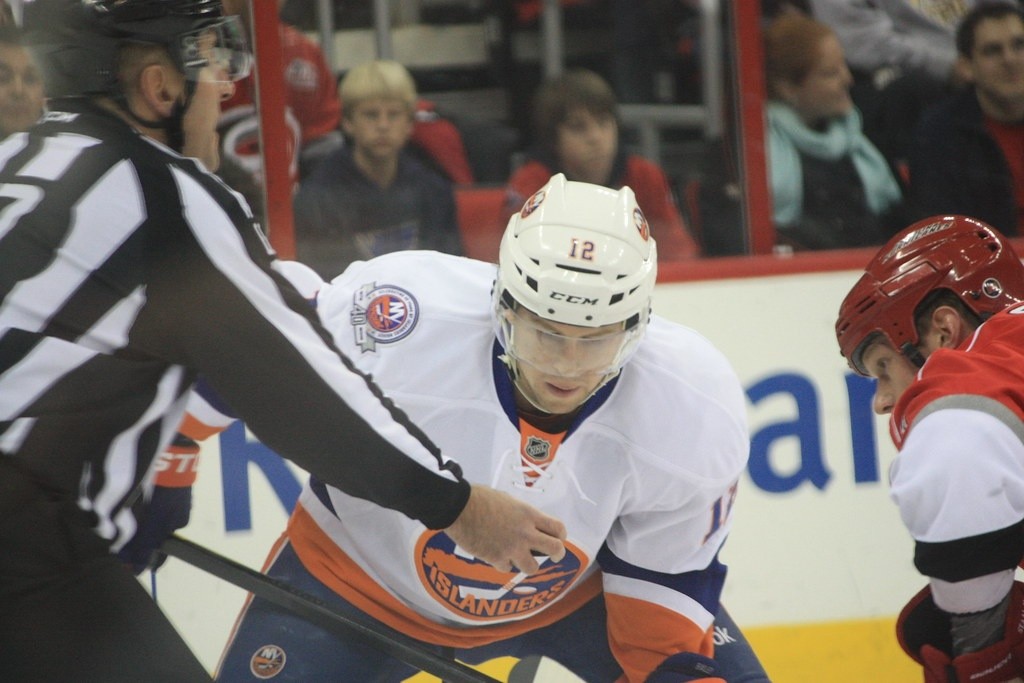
[118,429,200,574]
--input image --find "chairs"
[212,92,703,268]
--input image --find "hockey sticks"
[160,533,506,683]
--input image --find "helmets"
[489,172,657,340]
[836,215,1024,380]
[21,0,239,130]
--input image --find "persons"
[497,68,697,261]
[293,58,462,283]
[834,213,1024,683]
[763,0,1024,254]
[0,0,773,683]
